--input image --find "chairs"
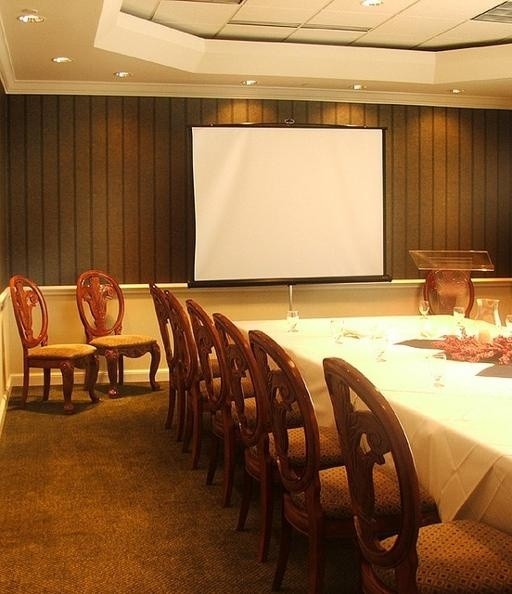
[323,358,510,594]
[425,268,476,320]
[246,330,437,593]
[8,269,162,416]
[150,282,306,484]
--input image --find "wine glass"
[286,311,302,334]
[454,305,467,329]
[417,300,431,323]
